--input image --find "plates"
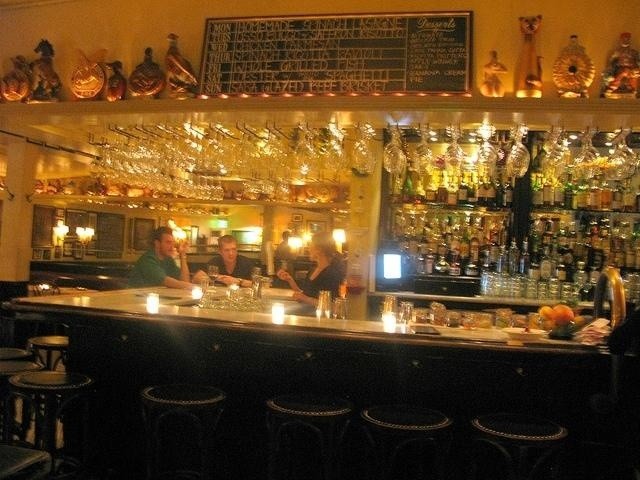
[500,328,546,340]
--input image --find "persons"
[600,32,640,99]
[273,231,292,271]
[191,234,258,288]
[276,233,342,307]
[125,227,208,291]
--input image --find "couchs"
[0,281,41,349]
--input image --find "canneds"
[626,251,635,269]
[552,216,561,232]
[541,216,551,229]
[542,230,553,247]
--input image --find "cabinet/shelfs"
[379,128,516,298]
[517,131,640,313]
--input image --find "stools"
[265,393,352,480]
[141,383,227,480]
[0,336,91,480]
[473,415,567,480]
[361,405,453,480]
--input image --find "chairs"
[32,279,58,297]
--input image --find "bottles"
[531,215,640,277]
[395,214,529,276]
[622,274,639,302]
[316,291,347,321]
[377,296,414,327]
[398,177,640,214]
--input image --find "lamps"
[52,220,70,246]
[76,226,94,249]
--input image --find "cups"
[479,275,580,301]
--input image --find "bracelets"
[238,278,242,287]
[180,255,187,259]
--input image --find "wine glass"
[249,266,261,291]
[209,264,219,290]
[93,123,173,203]
[176,124,227,204]
[295,121,376,185]
[384,122,638,178]
[234,127,291,204]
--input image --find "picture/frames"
[291,213,328,237]
[34,242,84,261]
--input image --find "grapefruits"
[554,303,575,327]
